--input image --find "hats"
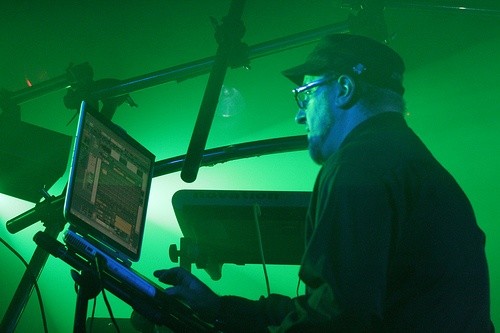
[281,33,406,96]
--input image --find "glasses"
[292,72,340,109]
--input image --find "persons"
[154,33,494,333]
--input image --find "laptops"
[62,99,195,316]
